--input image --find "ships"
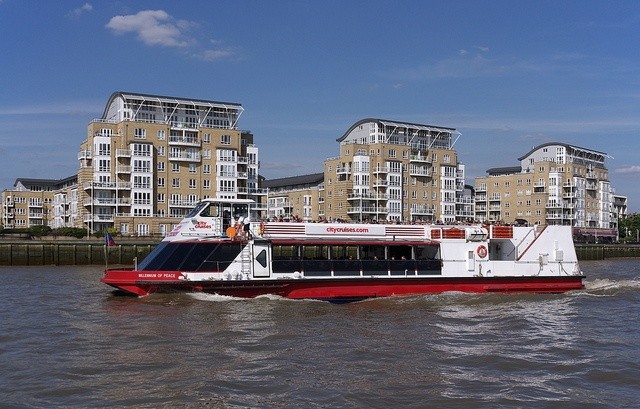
[98,197,587,300]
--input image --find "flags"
[106,232,117,246]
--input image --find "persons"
[346,254,410,260]
[219,210,253,240]
[264,213,381,223]
[382,217,435,225]
[441,219,529,227]
